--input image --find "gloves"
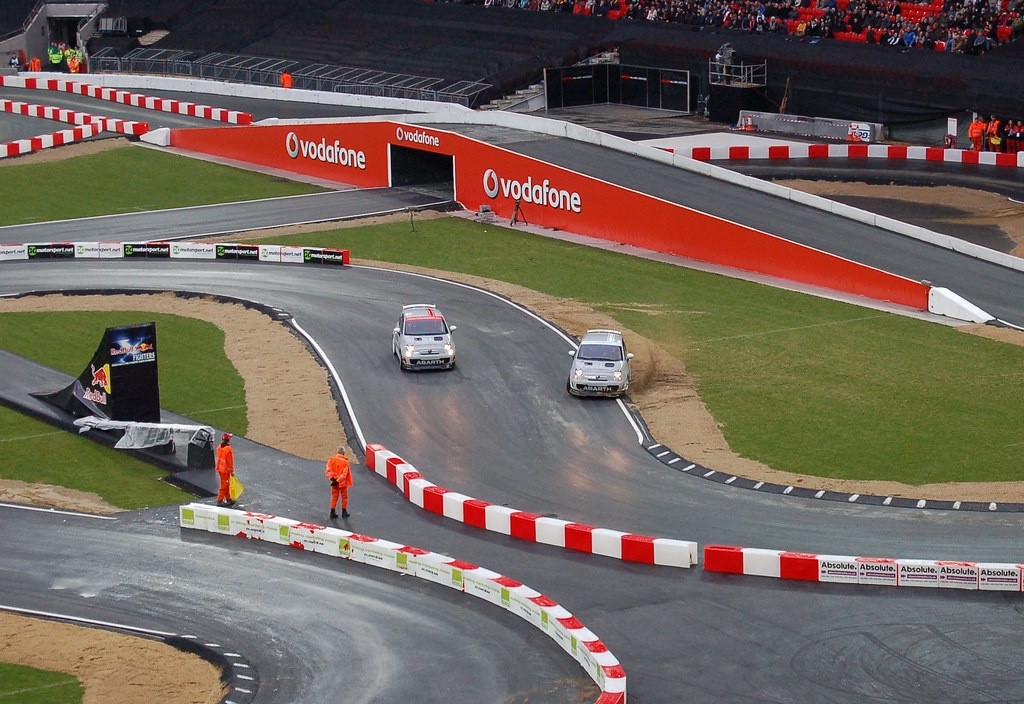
[331,478,336,481]
[330,481,338,486]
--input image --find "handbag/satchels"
[229,473,244,501]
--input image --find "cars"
[392,303,458,372]
[566,329,635,399]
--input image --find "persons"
[48,42,83,73]
[716,49,724,83]
[969,115,1024,153]
[215,433,236,506]
[325,447,352,518]
[435,0,621,17]
[281,70,293,88]
[29,56,41,72]
[625,0,1024,55]
[9,53,21,72]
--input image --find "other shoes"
[342,512,350,517]
[330,513,338,518]
[217,501,229,506]
[227,500,235,505]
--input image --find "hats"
[222,434,233,440]
